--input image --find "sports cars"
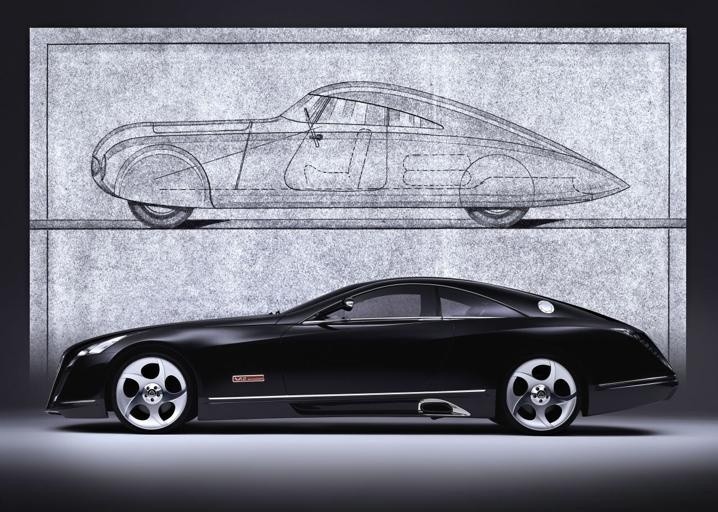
[88,82,632,227]
[45,276,680,434]
